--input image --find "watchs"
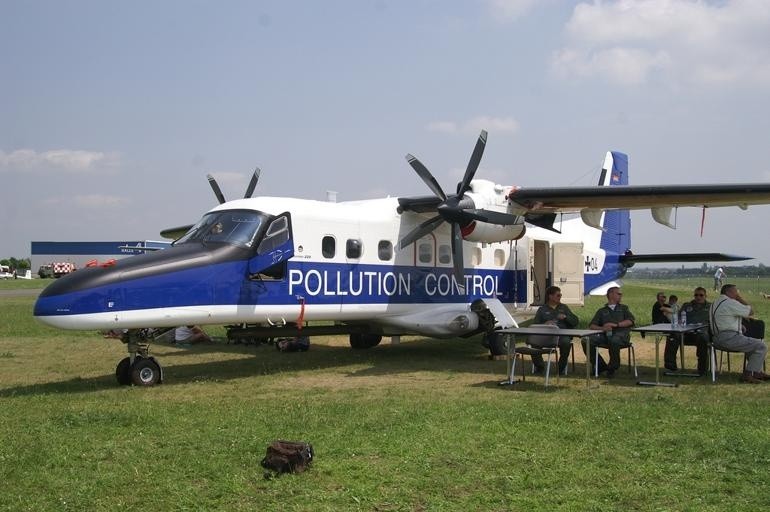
[563,314,567,319]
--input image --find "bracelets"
[613,320,620,328]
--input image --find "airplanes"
[26,127,769,386]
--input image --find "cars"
[0,272,13,281]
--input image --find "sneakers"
[557,361,565,374]
[535,365,545,375]
[594,364,615,377]
[740,371,770,384]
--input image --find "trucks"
[38,263,76,280]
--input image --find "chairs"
[510,324,561,389]
[717,317,766,382]
[594,343,637,379]
[555,342,576,372]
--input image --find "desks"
[633,322,707,389]
[492,326,604,391]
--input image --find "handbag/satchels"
[263,440,312,475]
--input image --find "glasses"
[694,294,704,296]
[553,294,562,297]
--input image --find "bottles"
[681,310,688,328]
[671,303,679,329]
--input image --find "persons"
[651,292,676,325]
[14,269,17,279]
[714,266,726,290]
[103,318,312,353]
[581,288,636,377]
[710,284,770,382]
[719,274,729,287]
[668,295,682,325]
[666,286,712,379]
[526,286,578,375]
